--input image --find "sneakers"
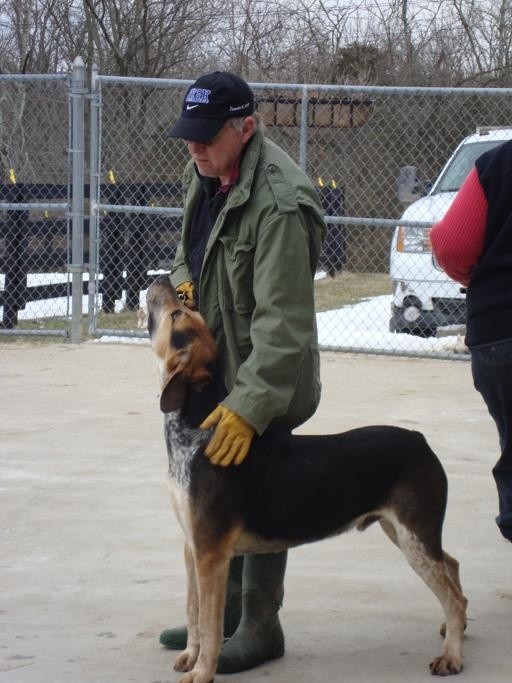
[215,613,287,674]
[158,594,244,651]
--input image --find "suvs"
[387,122,512,336]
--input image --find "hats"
[164,67,261,143]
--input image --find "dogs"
[145,273,469,682]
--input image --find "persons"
[429,139,512,549]
[158,72,330,675]
